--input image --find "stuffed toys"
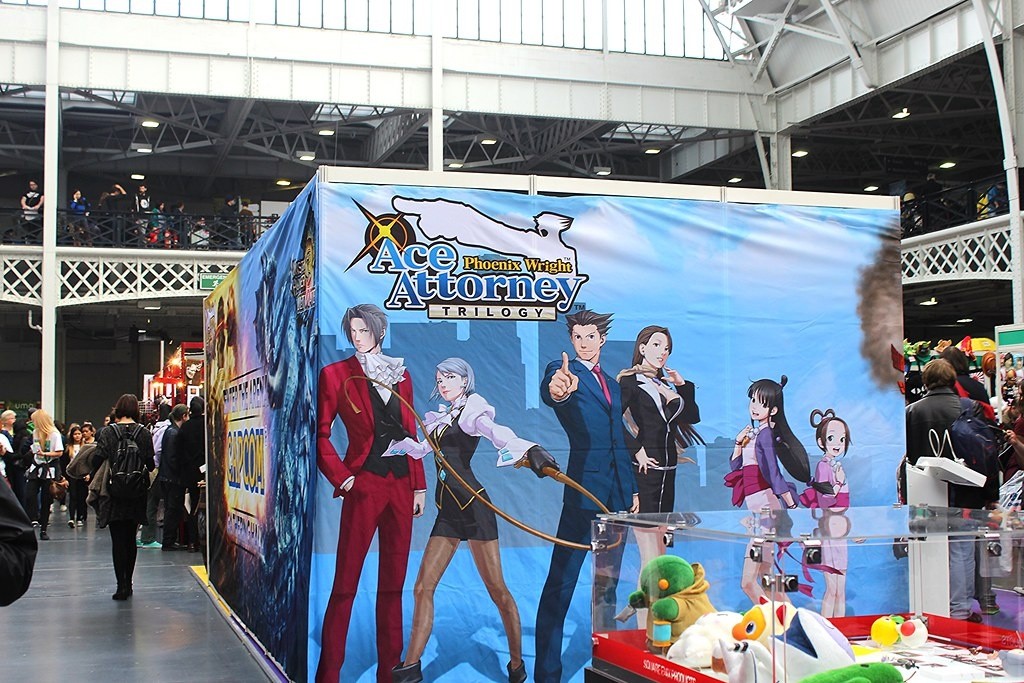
[613,555,928,683]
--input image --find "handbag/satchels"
[50,477,69,500]
[25,210,40,220]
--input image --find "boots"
[112,577,133,601]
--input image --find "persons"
[0,394,203,608]
[6,179,258,250]
[905,346,1024,621]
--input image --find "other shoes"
[982,605,1000,615]
[187,542,199,552]
[141,540,163,548]
[40,532,50,540]
[32,519,51,528]
[162,543,189,550]
[50,504,55,512]
[136,539,143,548]
[969,613,983,624]
[60,505,67,511]
[68,520,76,528]
[77,521,84,527]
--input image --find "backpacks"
[946,397,994,509]
[109,423,148,498]
